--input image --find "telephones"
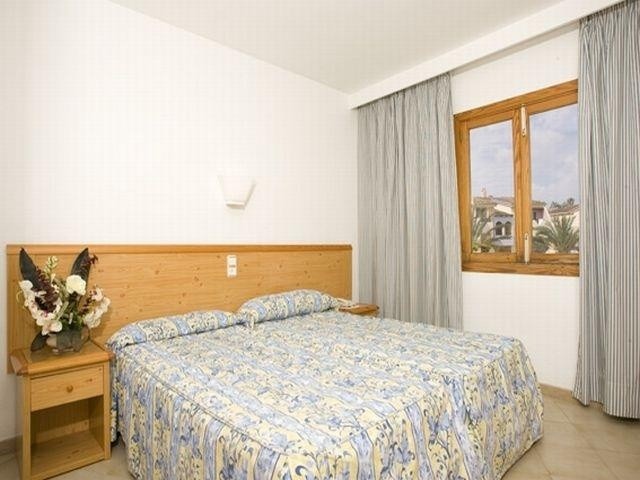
[337,298,353,307]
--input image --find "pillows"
[233,289,345,330]
[105,309,244,349]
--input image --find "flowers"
[16,247,110,354]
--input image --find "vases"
[45,332,74,354]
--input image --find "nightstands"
[10,340,116,480]
[340,301,379,318]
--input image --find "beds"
[6,244,544,480]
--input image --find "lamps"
[218,175,256,206]
